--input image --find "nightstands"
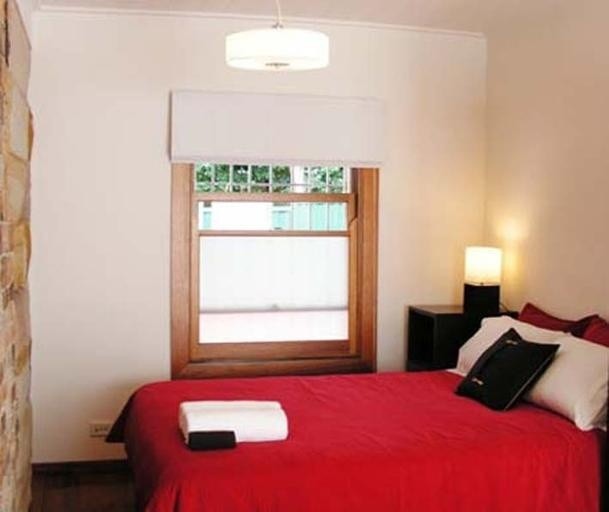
[405,306,518,371]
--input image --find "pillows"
[584,316,609,348]
[455,328,560,412]
[516,303,599,337]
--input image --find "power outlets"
[88,421,112,436]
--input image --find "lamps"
[463,245,501,312]
[225,1,330,72]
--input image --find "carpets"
[28,472,140,512]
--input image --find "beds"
[123,302,609,511]
